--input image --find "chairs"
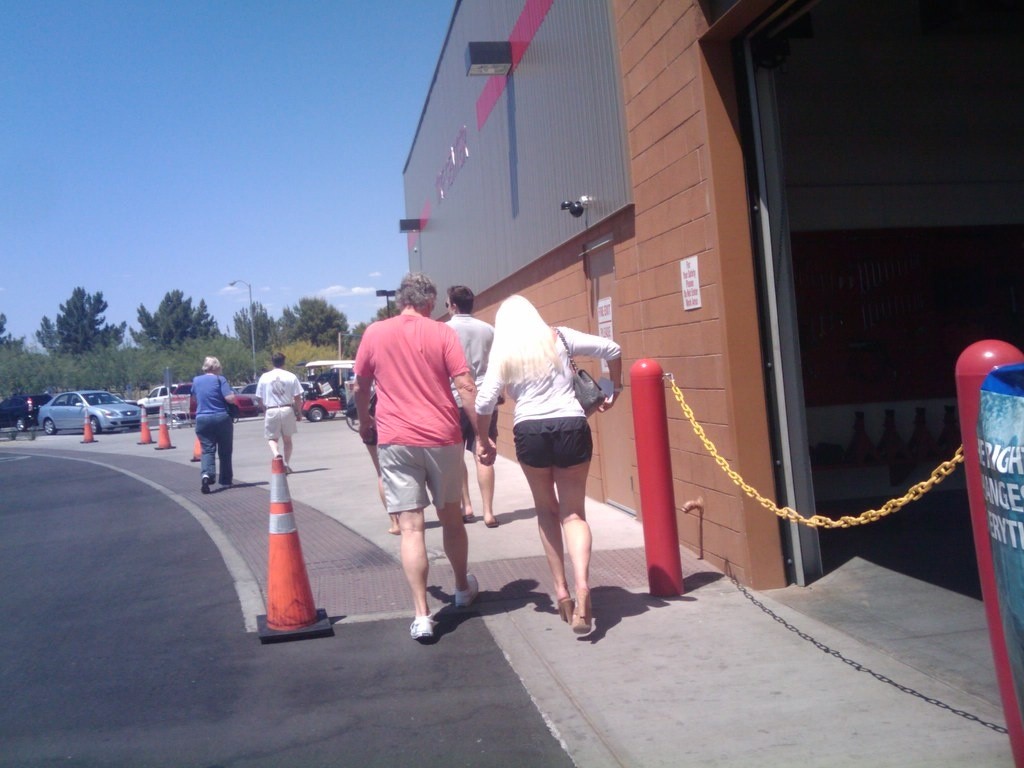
[73,398,79,406]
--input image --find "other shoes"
[223,483,233,489]
[275,454,282,458]
[284,465,292,473]
[201,475,210,494]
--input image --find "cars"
[135,385,171,414]
[169,378,344,424]
[36,389,143,435]
[0,392,57,432]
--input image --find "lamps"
[559,196,589,218]
[399,219,420,233]
[464,41,512,76]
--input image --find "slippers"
[487,517,499,527]
[463,515,476,523]
[388,528,401,535]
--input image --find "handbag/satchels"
[346,390,377,420]
[552,327,605,419]
[217,374,239,417]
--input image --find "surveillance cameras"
[560,201,584,218]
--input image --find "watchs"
[613,384,623,392]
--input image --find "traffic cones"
[153,405,177,451]
[136,407,157,445]
[256,455,336,644]
[80,410,99,443]
[190,435,202,462]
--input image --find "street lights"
[228,279,257,383]
[377,289,396,318]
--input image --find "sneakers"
[455,574,478,607]
[410,611,439,639]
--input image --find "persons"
[255,352,305,474]
[475,294,621,633]
[190,356,240,493]
[351,273,496,641]
[345,383,402,535]
[439,285,504,528]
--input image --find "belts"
[268,404,293,408]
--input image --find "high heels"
[558,597,575,624]
[571,588,592,634]
